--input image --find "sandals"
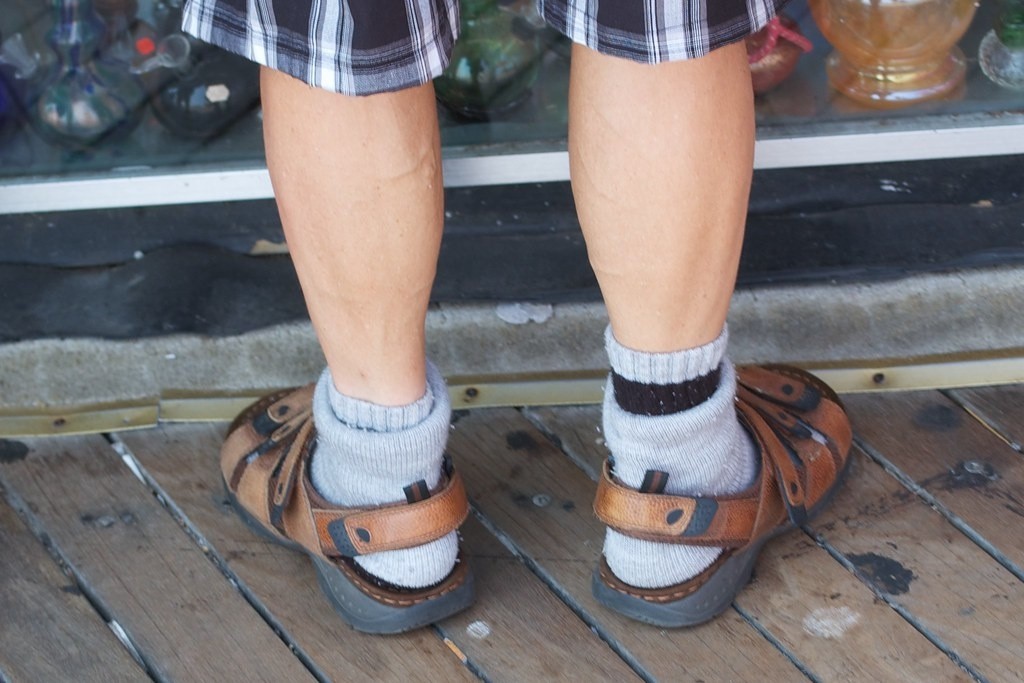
[593,361,855,628]
[219,384,481,634]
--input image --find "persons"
[181,0,857,637]
